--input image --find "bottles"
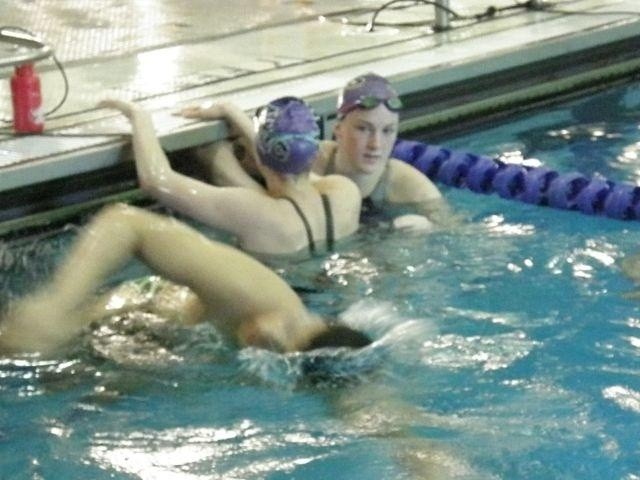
[9,60,44,136]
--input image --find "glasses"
[339,96,402,116]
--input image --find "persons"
[191,69,468,253]
[93,91,364,263]
[2,201,492,479]
